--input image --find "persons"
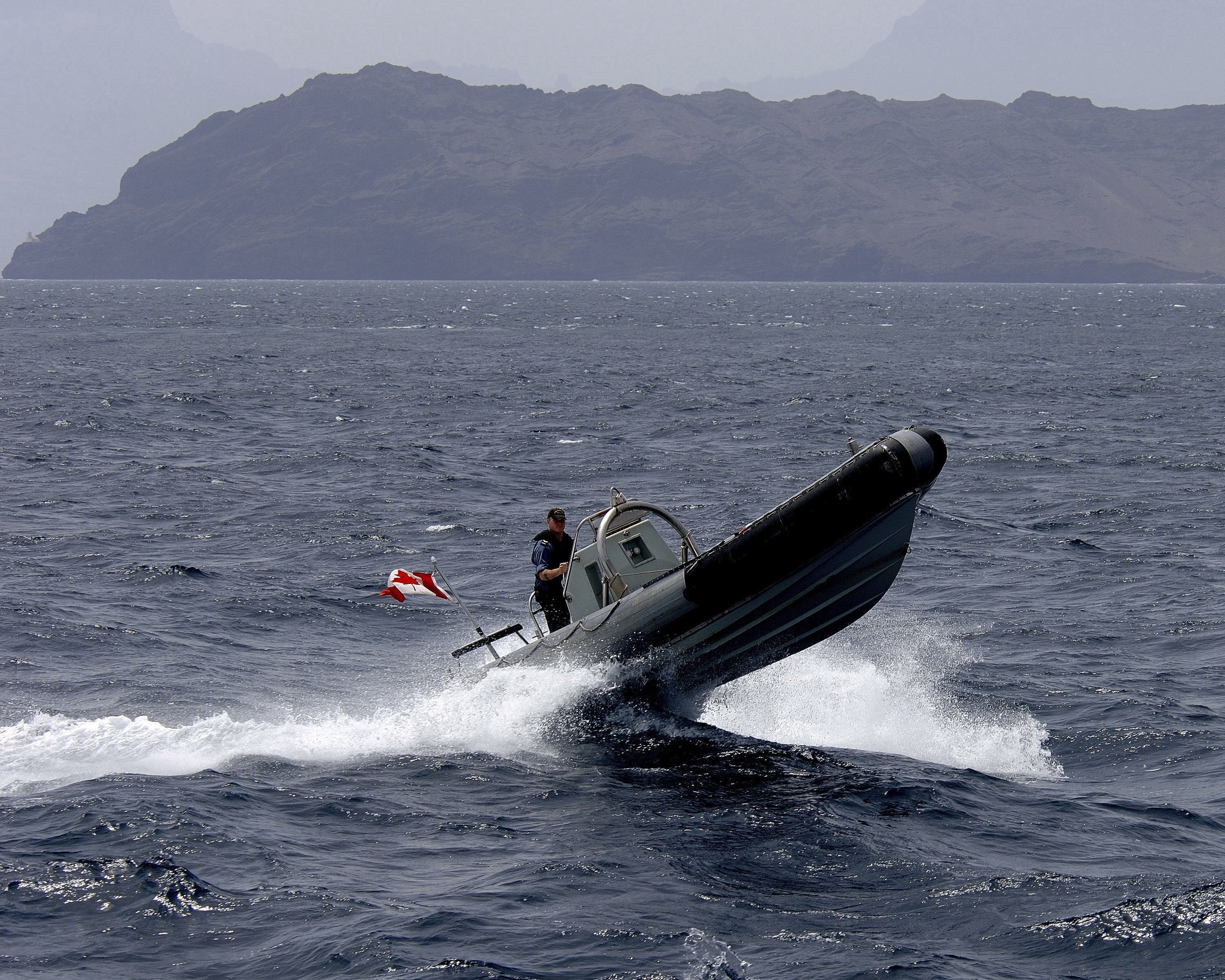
[531,508,578,634]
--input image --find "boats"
[448,422,948,720]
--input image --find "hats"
[547,508,567,520]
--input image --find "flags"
[379,568,454,603]
